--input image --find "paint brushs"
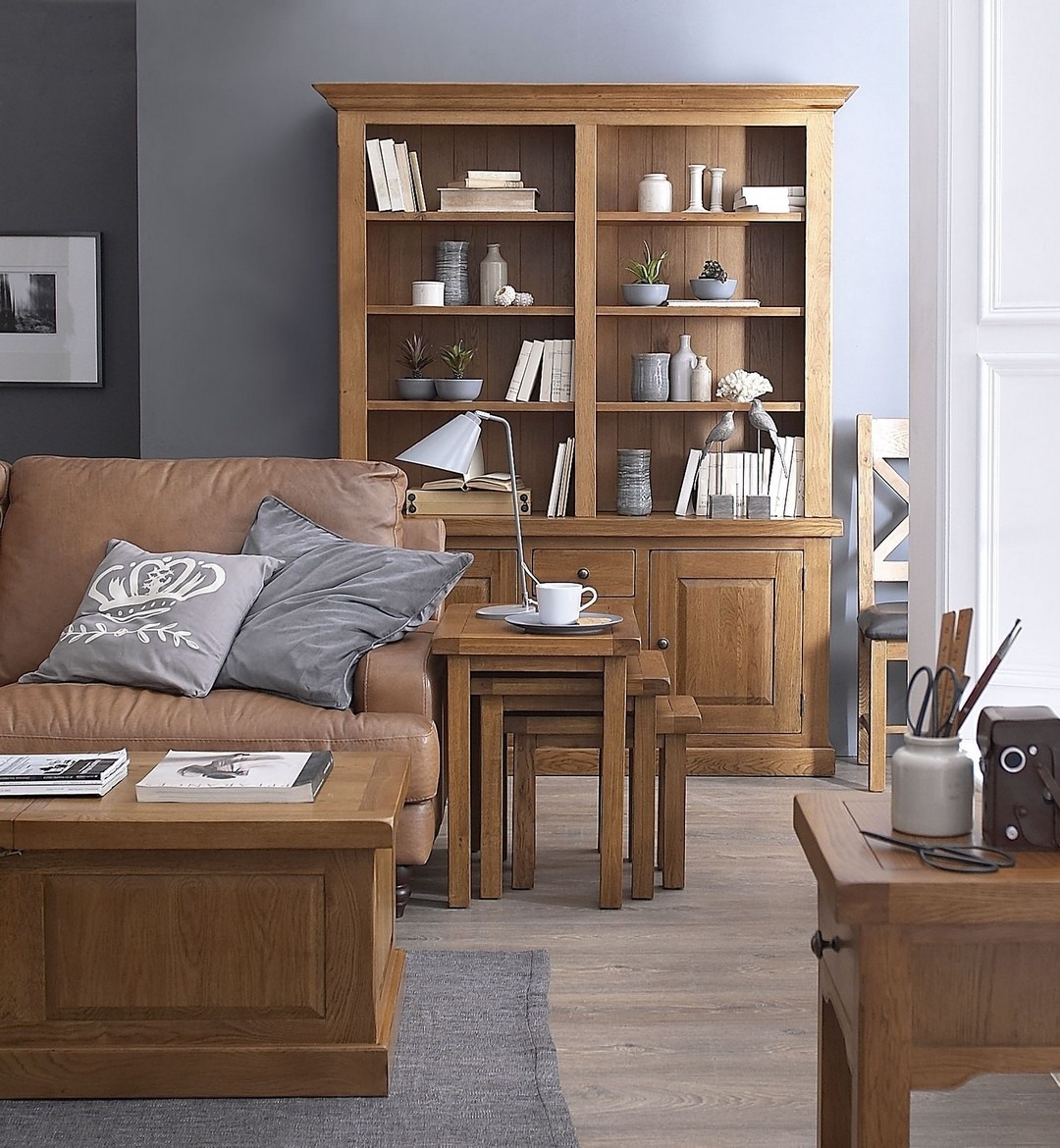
[948,618,1023,739]
[944,674,971,737]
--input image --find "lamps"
[394,410,537,620]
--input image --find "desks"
[791,792,1059,1147]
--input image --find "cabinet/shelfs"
[310,83,855,777]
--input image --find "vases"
[615,446,652,515]
[691,356,712,403]
[706,166,727,213]
[672,334,697,403]
[434,239,473,305]
[480,243,507,304]
[638,173,674,213]
[679,164,711,214]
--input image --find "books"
[504,335,575,404]
[545,436,576,519]
[362,135,543,212]
[733,183,807,212]
[134,747,334,804]
[0,747,131,796]
[675,433,805,518]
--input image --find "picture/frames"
[0,230,104,391]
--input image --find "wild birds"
[700,409,735,459]
[749,398,788,478]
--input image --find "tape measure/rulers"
[928,608,973,737]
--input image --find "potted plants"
[391,335,437,401]
[435,338,484,398]
[687,259,739,301]
[619,239,672,305]
[629,329,670,403]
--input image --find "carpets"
[1,950,583,1148]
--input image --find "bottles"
[670,334,697,402]
[892,730,974,837]
[637,173,672,212]
[480,243,508,306]
[691,356,712,402]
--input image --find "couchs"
[0,457,452,918]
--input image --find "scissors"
[904,665,959,737]
[862,831,1016,874]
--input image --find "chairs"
[855,413,909,792]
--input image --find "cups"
[536,582,598,624]
[435,240,470,306]
[616,448,652,515]
[630,353,671,402]
[411,281,445,307]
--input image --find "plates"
[504,613,624,634]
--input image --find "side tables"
[429,599,704,911]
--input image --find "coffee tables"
[3,752,414,1104]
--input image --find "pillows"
[212,496,475,714]
[16,536,285,705]
